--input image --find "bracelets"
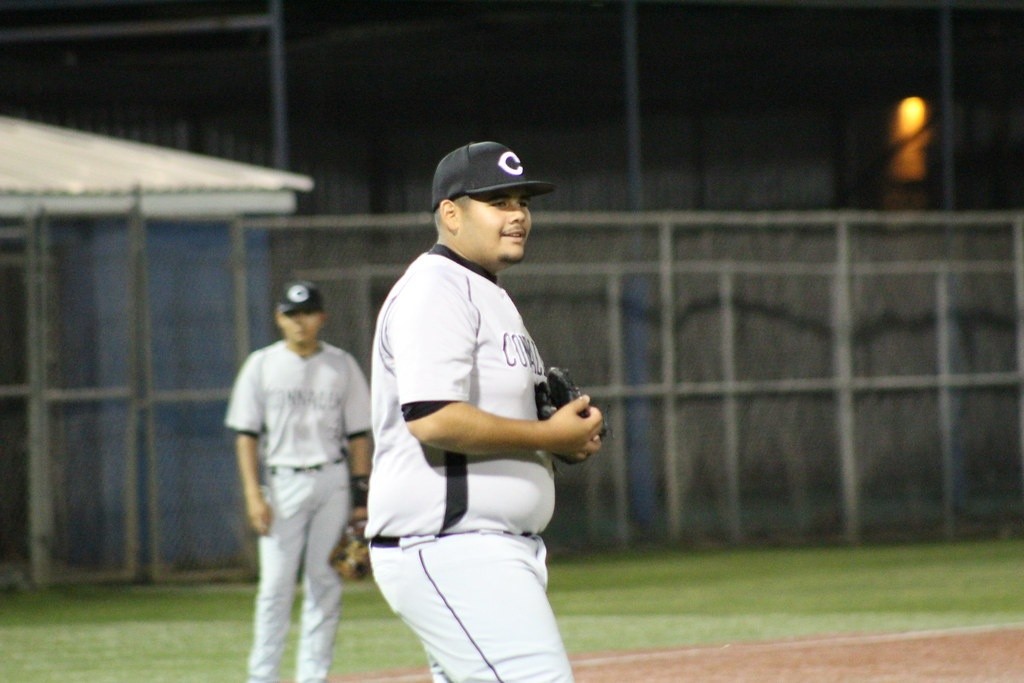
[350,475,369,508]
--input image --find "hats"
[432,142,556,212]
[278,281,324,313]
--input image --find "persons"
[225,280,372,683]
[364,142,603,682]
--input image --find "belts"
[370,531,532,547]
[269,457,344,475]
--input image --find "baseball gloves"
[534,365,608,466]
[327,514,371,581]
